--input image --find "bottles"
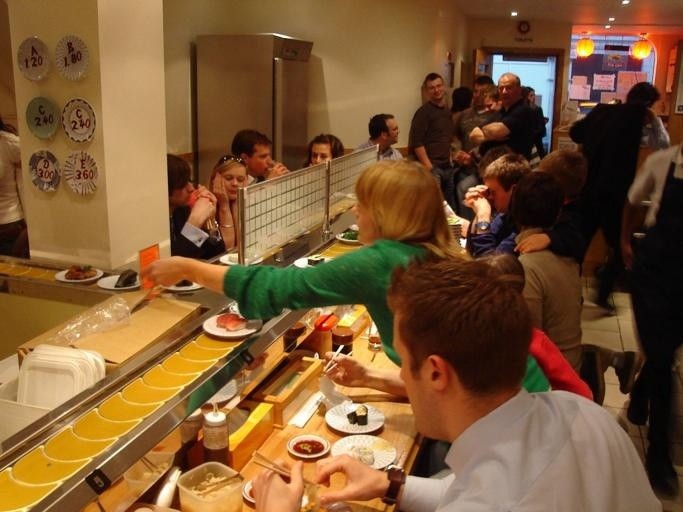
[203,401,230,467]
[332,327,353,357]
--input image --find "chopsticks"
[323,345,353,373]
[140,457,158,472]
[198,474,242,497]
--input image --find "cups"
[369,320,382,352]
[39,296,134,345]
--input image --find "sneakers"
[617,350,643,394]
[645,443,680,499]
[626,381,650,424]
[578,349,606,406]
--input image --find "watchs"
[381,464,407,506]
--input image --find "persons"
[167,153,226,261]
[0,114,29,260]
[409,72,549,238]
[462,82,683,501]
[303,133,345,168]
[138,156,552,482]
[353,114,404,161]
[250,252,663,512]
[232,129,290,183]
[207,154,249,252]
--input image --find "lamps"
[576,33,598,58]
[632,33,652,60]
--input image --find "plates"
[203,312,263,339]
[335,232,359,244]
[54,263,104,282]
[98,273,141,289]
[294,257,332,268]
[162,282,204,291]
[220,254,264,265]
[243,403,397,508]
[205,378,238,402]
[15,32,100,197]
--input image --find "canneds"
[332,327,353,354]
[284,323,307,353]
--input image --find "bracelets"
[220,223,235,228]
